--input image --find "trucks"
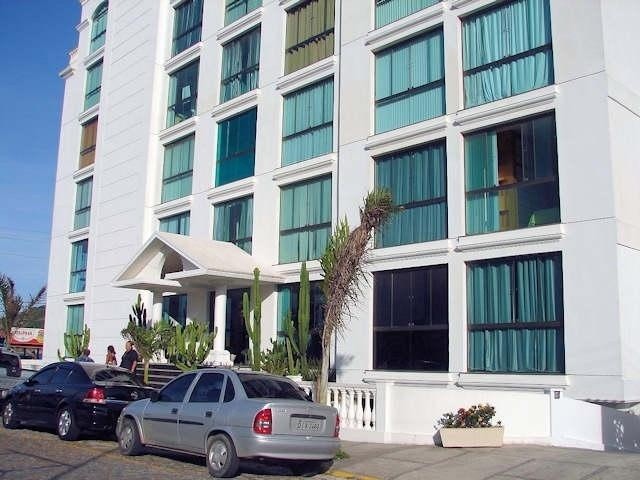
[0,350,40,391]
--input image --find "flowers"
[432,402,506,431]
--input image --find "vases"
[438,426,505,449]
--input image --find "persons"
[104,345,118,367]
[75,348,94,363]
[119,340,138,375]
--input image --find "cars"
[0,362,154,439]
[116,367,344,479]
